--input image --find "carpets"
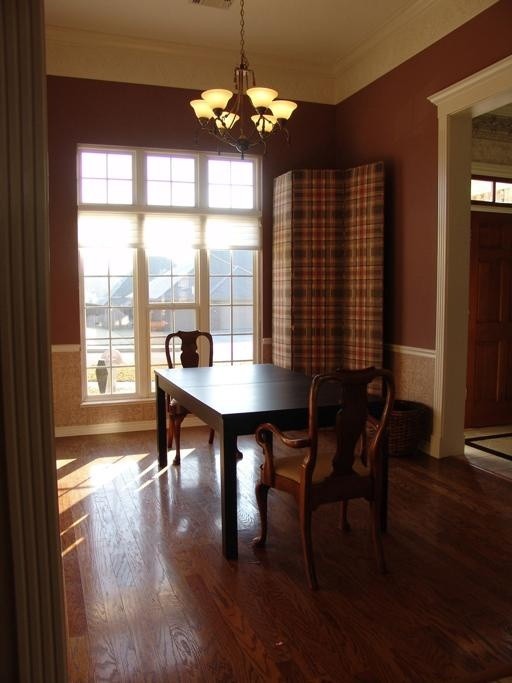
[464,424,512,481]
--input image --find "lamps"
[190,2,299,160]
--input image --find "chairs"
[165,330,243,465]
[250,366,395,590]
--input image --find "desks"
[153,363,388,560]
